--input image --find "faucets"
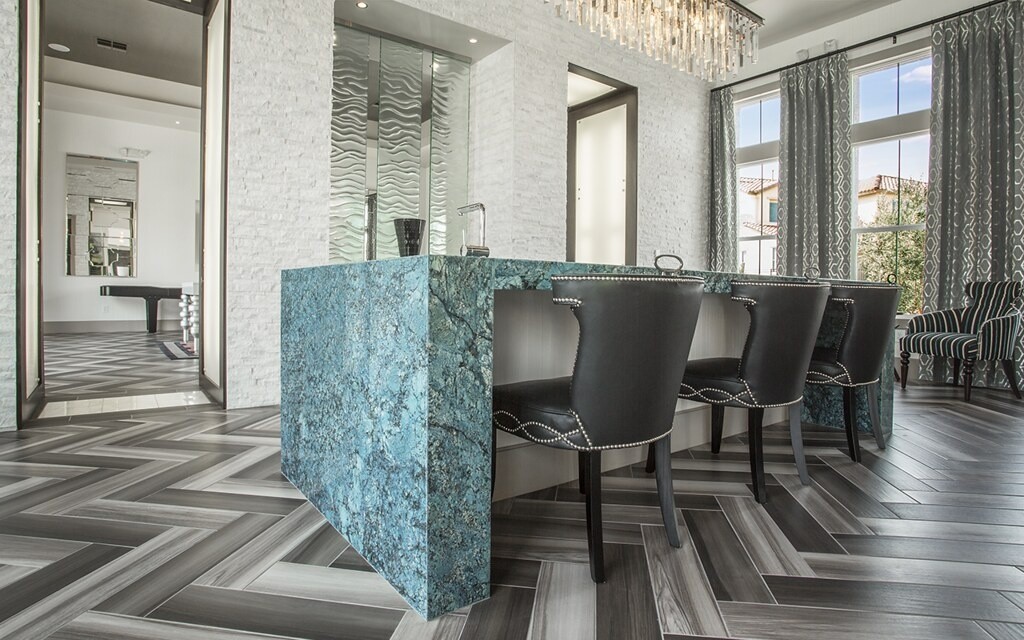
[456,201,490,257]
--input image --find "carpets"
[156,339,199,361]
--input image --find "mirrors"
[63,151,139,278]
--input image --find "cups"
[394,218,426,258]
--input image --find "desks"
[179,281,199,353]
[99,285,182,332]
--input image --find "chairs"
[644,280,831,504]
[760,284,904,464]
[899,280,1024,403]
[491,275,708,584]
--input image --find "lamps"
[544,0,765,86]
[121,147,146,157]
